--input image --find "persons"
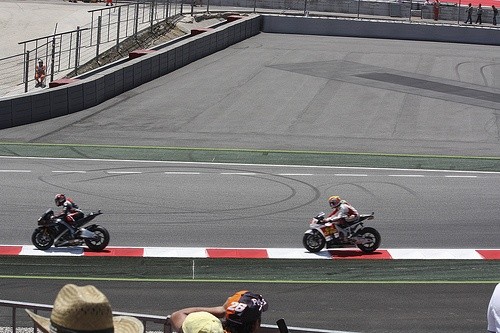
[178,311,224,333]
[323,196,359,238]
[50,194,84,244]
[425,0,430,4]
[492,5,498,25]
[170,290,268,333]
[432,0,440,21]
[464,3,474,24]
[35,59,46,83]
[475,3,483,24]
[25,283,144,333]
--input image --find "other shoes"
[66,233,77,240]
[35,83,45,88]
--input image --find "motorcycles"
[31,208,111,252]
[303,212,381,252]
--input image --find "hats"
[329,196,341,208]
[180,311,224,333]
[25,284,145,333]
[223,290,269,327]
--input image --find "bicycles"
[34,68,46,88]
